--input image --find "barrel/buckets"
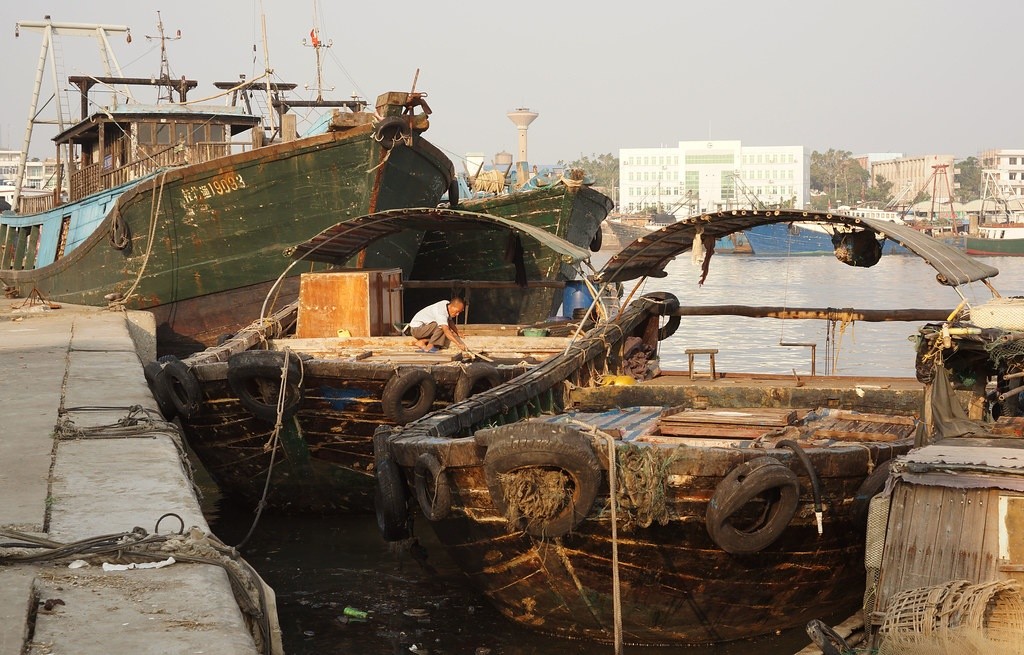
[523,328,550,337]
[562,280,598,319]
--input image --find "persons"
[410,297,469,353]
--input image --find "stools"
[685,349,718,382]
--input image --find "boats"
[0,7,617,354]
[604,183,755,254]
[740,163,967,256]
[142,206,1024,655]
[966,170,1024,257]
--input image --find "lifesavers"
[704,455,799,557]
[452,360,503,407]
[145,359,176,424]
[381,368,438,425]
[226,349,310,424]
[157,354,204,419]
[371,426,406,541]
[412,453,450,521]
[852,460,902,522]
[484,422,602,541]
[642,292,681,341]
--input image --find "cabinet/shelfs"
[295,268,405,338]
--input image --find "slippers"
[427,347,439,353]
[414,350,425,353]
